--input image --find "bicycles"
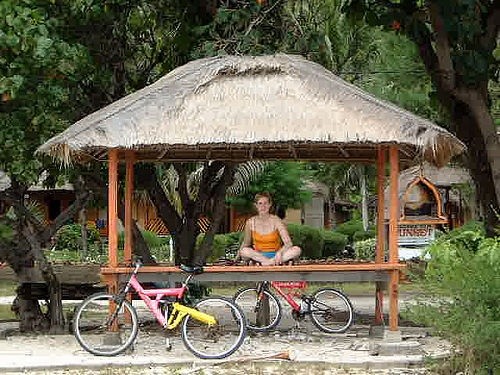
[231,257,354,334]
[72,253,247,361]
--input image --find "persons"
[240,191,302,266]
